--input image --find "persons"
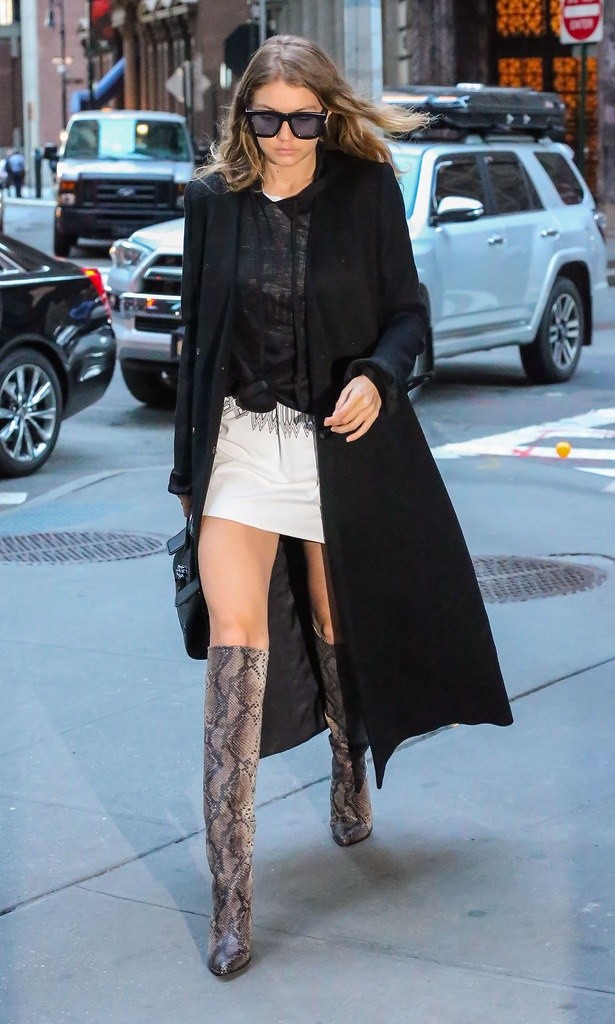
[0,147,42,199]
[168,32,512,979]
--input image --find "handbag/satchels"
[167,499,210,660]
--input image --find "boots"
[312,623,373,847]
[203,646,270,976]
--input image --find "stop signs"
[559,0,604,45]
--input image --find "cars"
[0,230,116,479]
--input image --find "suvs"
[107,80,612,411]
[53,108,198,256]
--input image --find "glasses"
[245,104,328,139]
